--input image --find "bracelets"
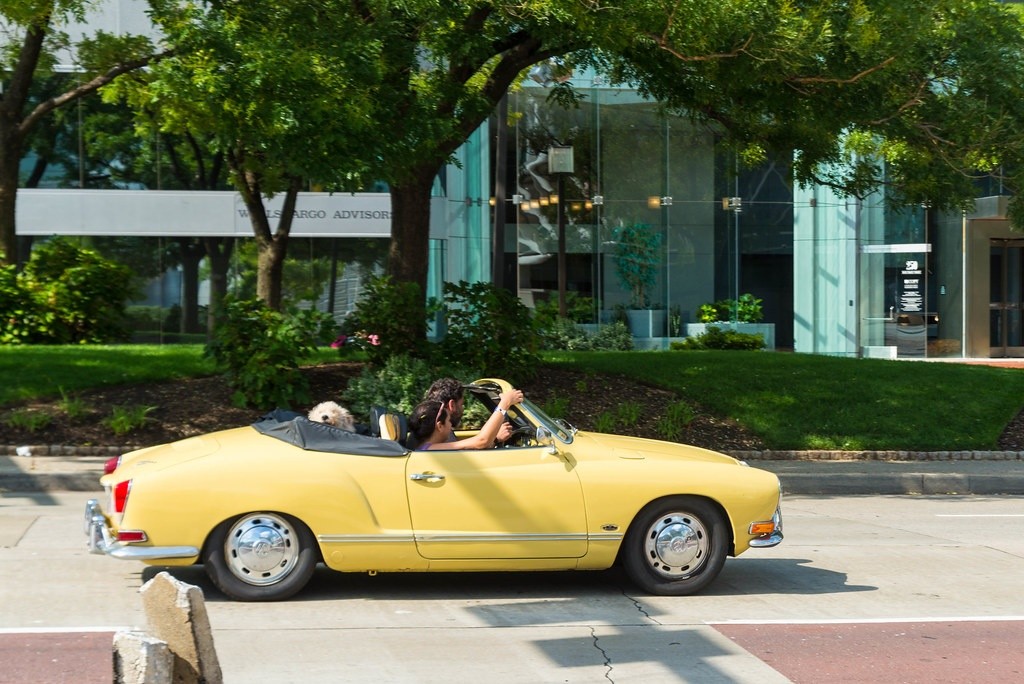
[493,438,500,444]
[496,407,508,417]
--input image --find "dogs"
[307,401,357,433]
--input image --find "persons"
[404,388,524,451]
[424,378,513,450]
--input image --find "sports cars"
[85,376,784,603]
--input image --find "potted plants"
[612,218,674,337]
[683,293,777,352]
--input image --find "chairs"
[369,404,408,448]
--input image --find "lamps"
[520,193,737,214]
